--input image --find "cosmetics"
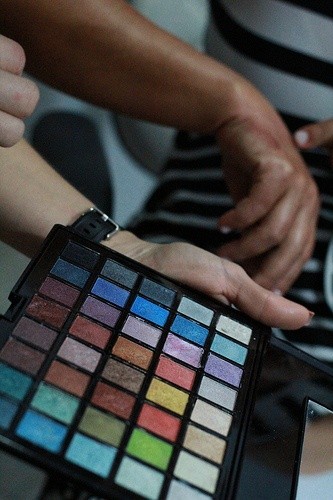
[2,225,333,500]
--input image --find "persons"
[0,33,315,330]
[1,0,333,366]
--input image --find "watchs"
[69,206,124,246]
